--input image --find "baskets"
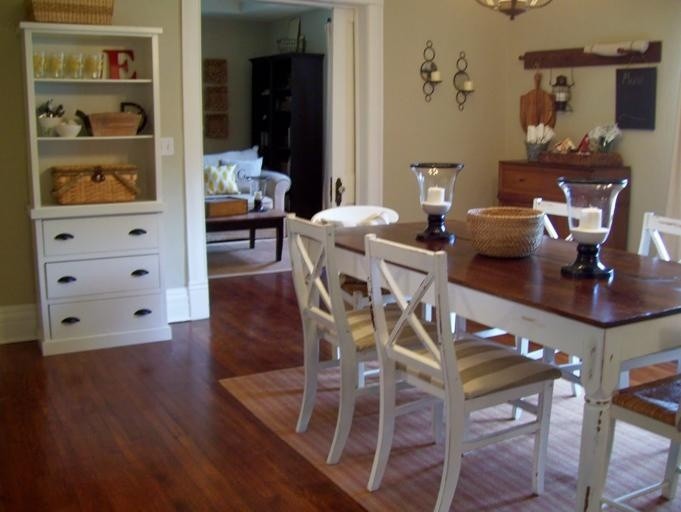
[467,205,545,258]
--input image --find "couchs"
[205,146,293,212]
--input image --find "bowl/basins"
[56,125,83,138]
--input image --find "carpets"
[208,237,295,279]
[220,357,680,512]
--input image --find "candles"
[425,185,446,205]
[579,203,602,231]
[464,80,474,91]
[432,70,441,81]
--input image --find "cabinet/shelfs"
[498,160,629,251]
[202,57,229,140]
[249,52,323,219]
[519,41,663,68]
[18,24,173,356]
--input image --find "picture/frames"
[613,70,656,130]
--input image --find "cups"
[50,49,64,79]
[88,50,102,79]
[34,49,46,78]
[69,50,84,79]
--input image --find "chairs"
[362,233,563,511]
[602,360,680,511]
[638,210,679,259]
[312,205,398,307]
[286,211,438,466]
[507,197,585,419]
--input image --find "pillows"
[207,165,240,195]
[231,159,264,195]
[205,145,259,167]
